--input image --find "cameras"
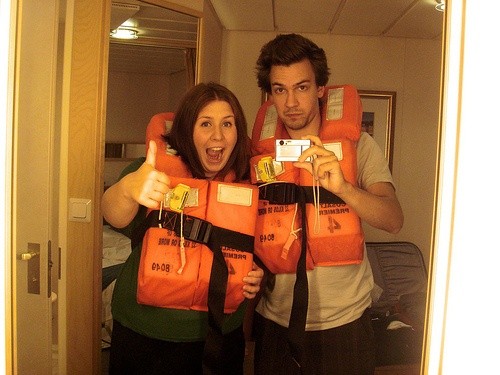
[275,138,311,161]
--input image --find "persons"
[101,82,264,375]
[247,33,405,375]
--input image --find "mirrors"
[0,0,462,375]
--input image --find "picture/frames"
[357,89,396,177]
